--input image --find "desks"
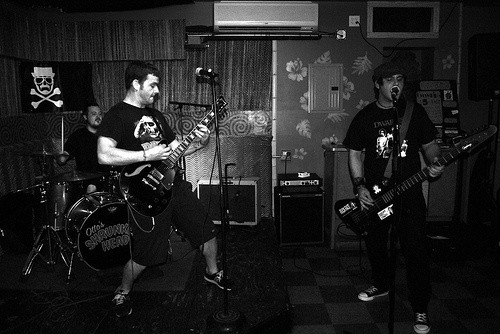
[322,145,428,250]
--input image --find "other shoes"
[204,269,236,291]
[113,293,133,317]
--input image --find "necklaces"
[377,99,393,108]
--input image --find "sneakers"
[357,286,390,302]
[413,312,431,334]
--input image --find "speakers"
[274,186,327,244]
[198,176,261,226]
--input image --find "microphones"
[390,85,400,96]
[195,67,219,79]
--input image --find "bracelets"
[143,150,147,162]
[351,176,365,189]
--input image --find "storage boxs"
[197,177,261,225]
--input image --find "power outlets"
[279,151,291,161]
[336,29,346,40]
[349,15,360,27]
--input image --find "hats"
[374,62,408,78]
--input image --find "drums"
[65,191,144,272]
[34,175,69,232]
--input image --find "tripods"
[21,149,68,272]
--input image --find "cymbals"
[48,172,102,183]
[15,152,76,160]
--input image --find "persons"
[342,63,444,334]
[51,60,235,318]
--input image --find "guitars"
[118,96,229,219]
[334,124,498,239]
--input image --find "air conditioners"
[213,0,318,34]
